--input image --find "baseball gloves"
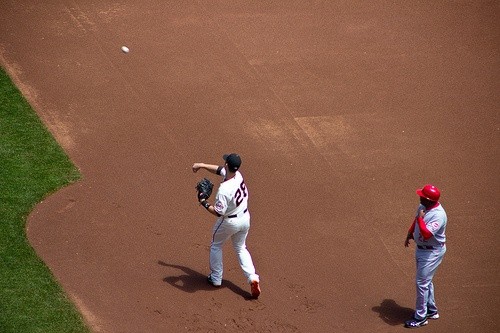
[197,178,213,202]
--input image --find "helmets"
[416,184,440,201]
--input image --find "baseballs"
[121,45,129,53]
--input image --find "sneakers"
[427,309,439,318]
[251,281,260,296]
[406,317,428,328]
[208,276,221,286]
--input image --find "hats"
[223,153,241,167]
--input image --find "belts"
[228,209,247,217]
[416,243,441,249]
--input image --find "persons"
[193,153,261,298]
[404,184,448,328]
